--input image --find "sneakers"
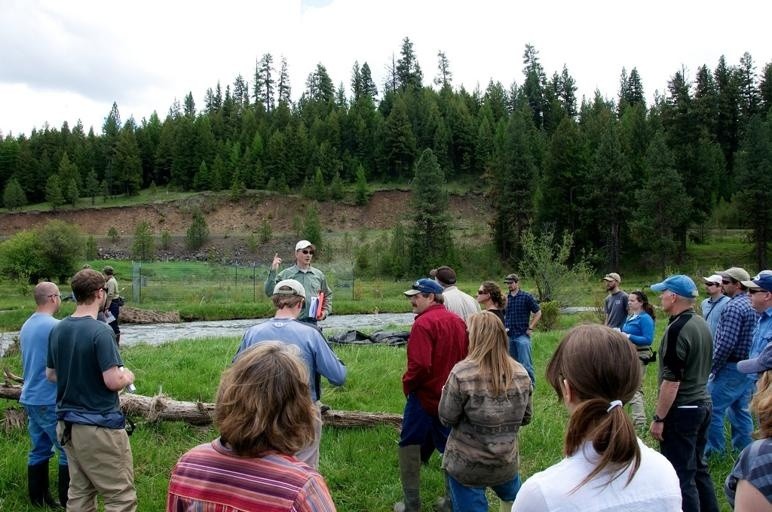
[316,400,330,413]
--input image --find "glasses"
[705,282,717,286]
[299,250,312,255]
[722,279,731,285]
[102,287,109,293]
[478,290,488,295]
[48,294,62,297]
[749,288,768,294]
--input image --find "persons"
[699,266,772,468]
[510,323,683,512]
[231,277,348,473]
[166,339,339,512]
[17,280,71,510]
[45,267,138,512]
[394,266,541,512]
[102,265,123,346]
[72,264,93,304]
[724,370,772,512]
[649,274,719,512]
[263,239,335,415]
[602,273,655,432]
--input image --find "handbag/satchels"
[118,297,124,306]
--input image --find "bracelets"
[105,307,109,310]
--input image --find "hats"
[715,267,750,283]
[404,279,443,296]
[603,272,621,283]
[104,266,114,275]
[430,266,456,284]
[737,342,772,374]
[504,274,519,282]
[273,279,307,309]
[296,240,315,251]
[651,274,698,298]
[704,275,722,285]
[740,270,772,291]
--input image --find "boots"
[58,464,70,508]
[28,459,60,509]
[394,444,421,512]
[437,453,450,512]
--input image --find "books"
[315,291,324,319]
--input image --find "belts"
[637,347,653,351]
